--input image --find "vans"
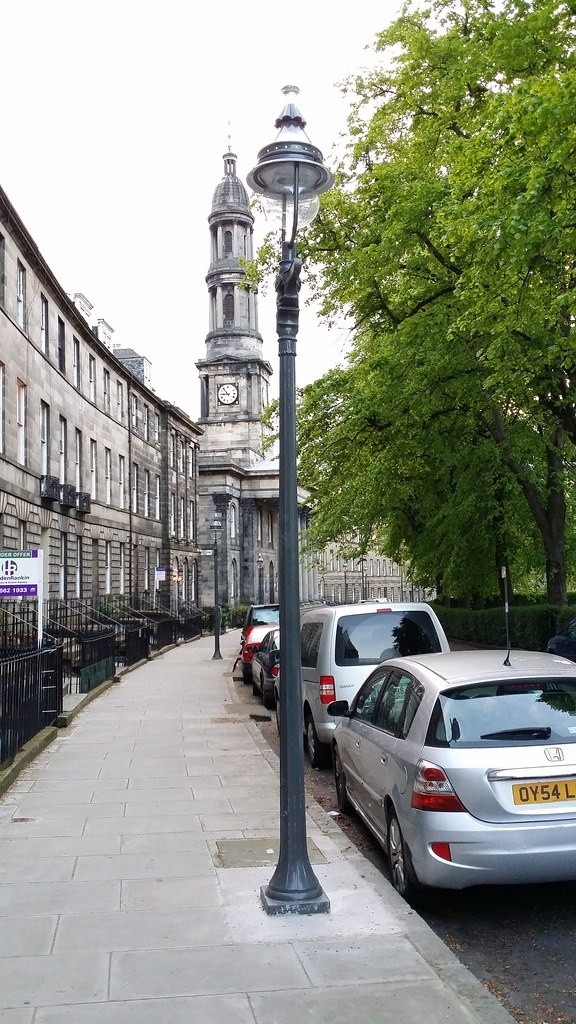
[267,598,453,769]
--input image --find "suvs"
[238,603,280,684]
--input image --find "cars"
[325,648,576,900]
[247,628,281,711]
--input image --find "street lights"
[363,564,367,601]
[244,78,329,917]
[343,561,349,605]
[207,515,225,662]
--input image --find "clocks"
[216,382,240,405]
